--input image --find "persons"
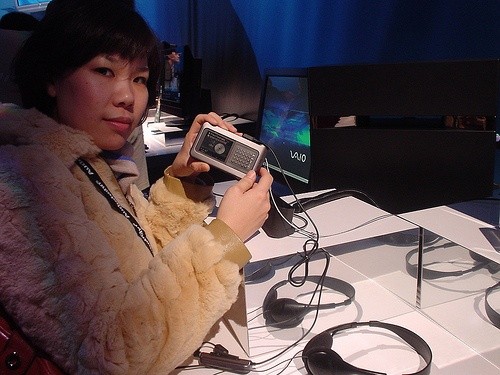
[0,1,273,375]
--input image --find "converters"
[200,352,252,374]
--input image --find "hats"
[162,40,177,50]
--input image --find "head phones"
[302,321,432,375]
[405,242,500,279]
[263,274,355,329]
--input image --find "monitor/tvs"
[253,66,318,184]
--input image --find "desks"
[168,181,500,375]
[139,109,186,157]
